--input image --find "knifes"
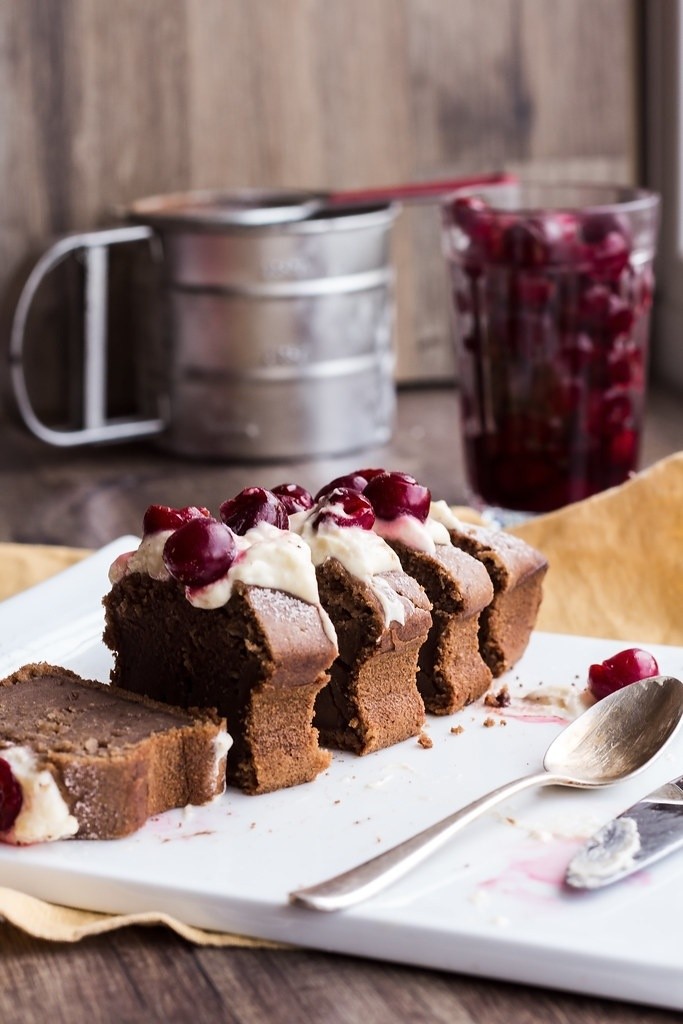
[562,774,683,891]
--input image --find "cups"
[433,180,661,515]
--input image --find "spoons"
[288,676,683,914]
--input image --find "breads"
[0,466,548,844]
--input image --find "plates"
[0,534,683,1010]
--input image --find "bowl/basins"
[122,183,400,461]
